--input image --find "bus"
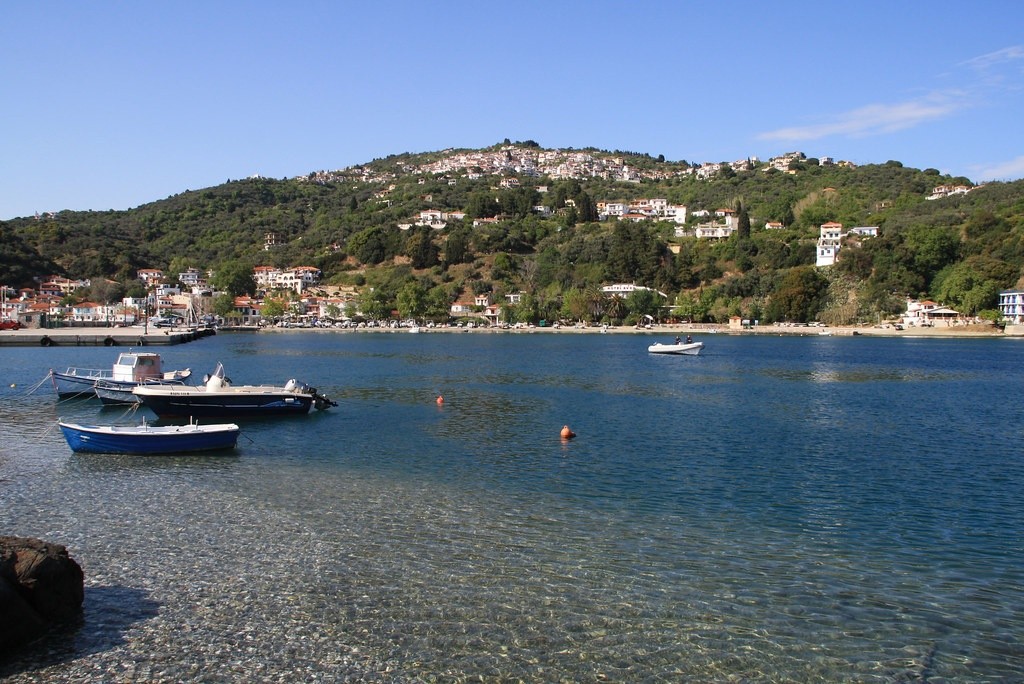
[775,320,828,327]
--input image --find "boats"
[130,363,340,417]
[819,331,833,336]
[49,350,192,401]
[94,379,232,408]
[180,298,218,329]
[56,420,240,457]
[647,342,704,356]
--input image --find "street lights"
[48,298,53,328]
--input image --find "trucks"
[539,320,552,327]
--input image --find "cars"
[245,314,537,328]
[0,320,23,331]
[895,325,903,330]
[153,320,177,330]
